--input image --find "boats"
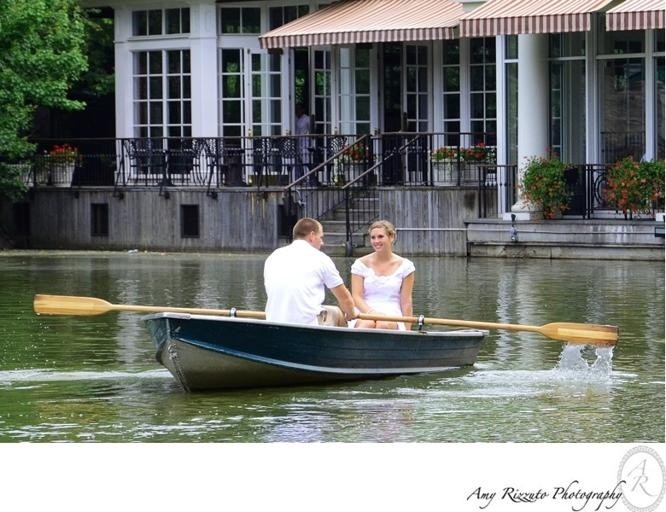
[140,310,490,392]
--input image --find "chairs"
[124,135,347,187]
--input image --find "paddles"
[33,293,266,319]
[345,307,618,347]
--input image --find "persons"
[263,216,360,327]
[295,101,311,182]
[344,219,416,330]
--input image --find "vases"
[48,157,76,188]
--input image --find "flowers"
[347,143,370,160]
[601,154,665,215]
[47,143,78,158]
[513,153,576,218]
[430,143,496,164]
